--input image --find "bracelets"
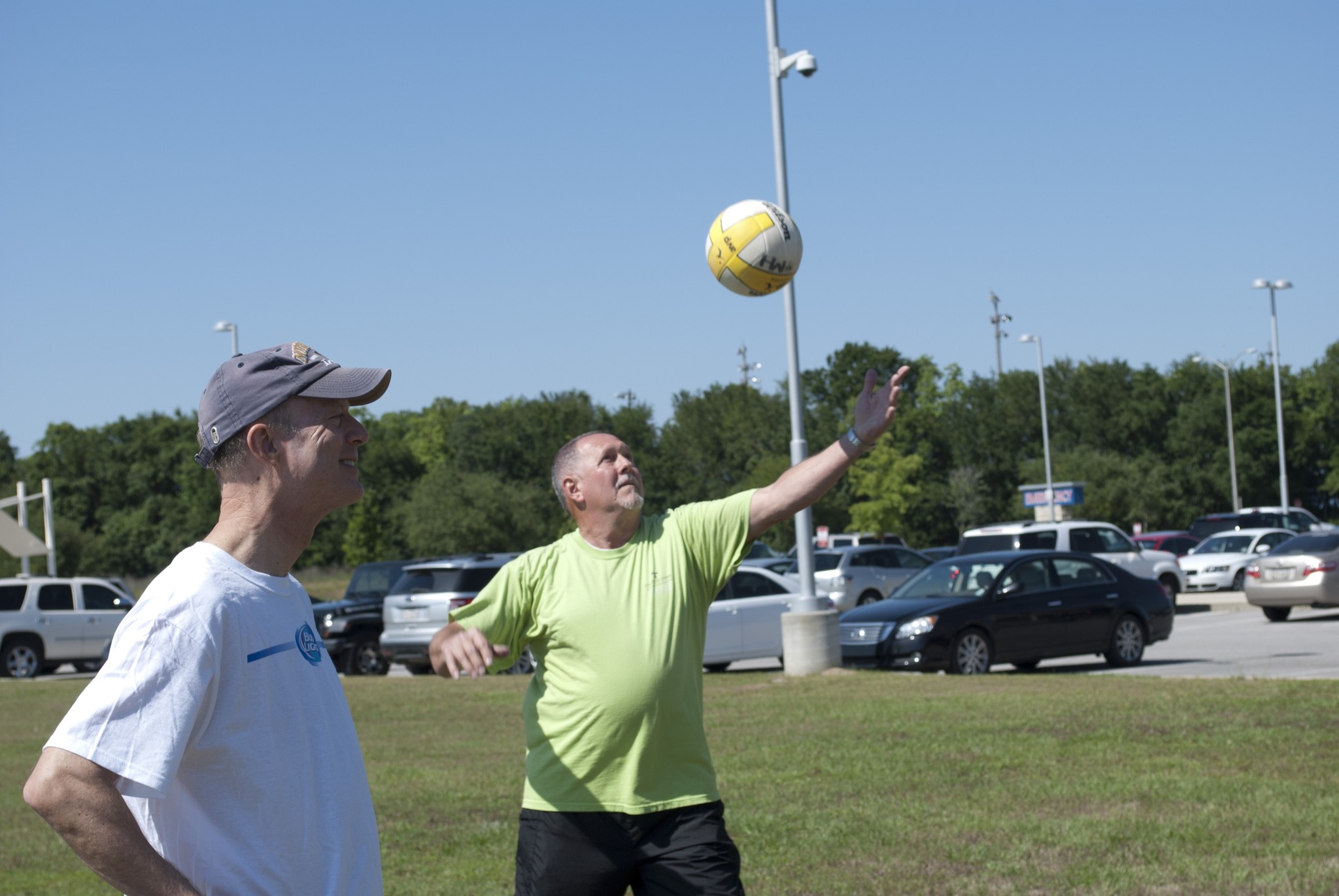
[846,429,877,453]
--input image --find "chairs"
[976,572,992,587]
[1077,568,1093,578]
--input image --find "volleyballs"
[704,198,803,299]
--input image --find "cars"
[703,564,833,672]
[0,571,136,679]
[1126,529,1203,558]
[836,548,1175,676]
[1243,528,1339,623]
[1176,528,1300,593]
[740,532,957,612]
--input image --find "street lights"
[1018,333,1056,523]
[1191,346,1259,514]
[1251,276,1296,530]
[213,320,240,357]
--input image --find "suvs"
[945,518,1187,598]
[1191,505,1339,534]
[311,550,450,676]
[377,550,537,676]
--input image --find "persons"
[21,343,393,896]
[430,363,912,896]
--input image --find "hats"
[194,342,392,467]
